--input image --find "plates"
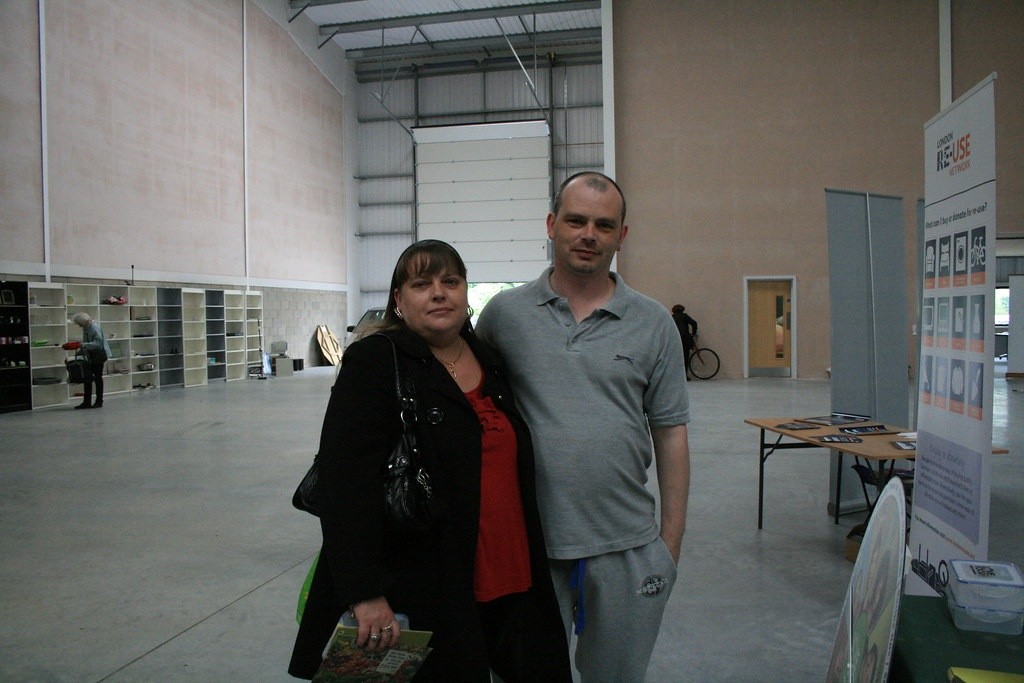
[136,317,150,320]
[133,334,153,337]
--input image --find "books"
[310,623,434,683]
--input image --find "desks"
[316,325,343,365]
[887,595,1024,683]
[744,416,918,529]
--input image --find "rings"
[384,624,391,633]
[371,633,380,640]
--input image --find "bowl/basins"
[949,560,1024,612]
[947,589,1023,635]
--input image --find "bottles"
[262,353,272,375]
[67,294,75,304]
[130,307,134,320]
[973,303,981,334]
[31,314,49,325]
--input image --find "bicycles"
[689,333,720,380]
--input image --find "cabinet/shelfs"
[0,280,265,414]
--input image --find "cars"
[342,306,390,359]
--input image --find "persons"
[73,313,111,410]
[672,304,697,380]
[474,171,692,683]
[289,240,574,683]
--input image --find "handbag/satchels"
[66,347,93,383]
[88,348,107,364]
[292,333,436,535]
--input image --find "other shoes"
[75,401,91,409]
[91,399,103,407]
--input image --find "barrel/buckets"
[293,359,304,371]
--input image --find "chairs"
[847,465,913,542]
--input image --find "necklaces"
[435,340,464,379]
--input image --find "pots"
[137,362,155,371]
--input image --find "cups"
[19,361,26,367]
[10,361,16,368]
[29,294,37,304]
[22,336,28,343]
[0,337,6,344]
